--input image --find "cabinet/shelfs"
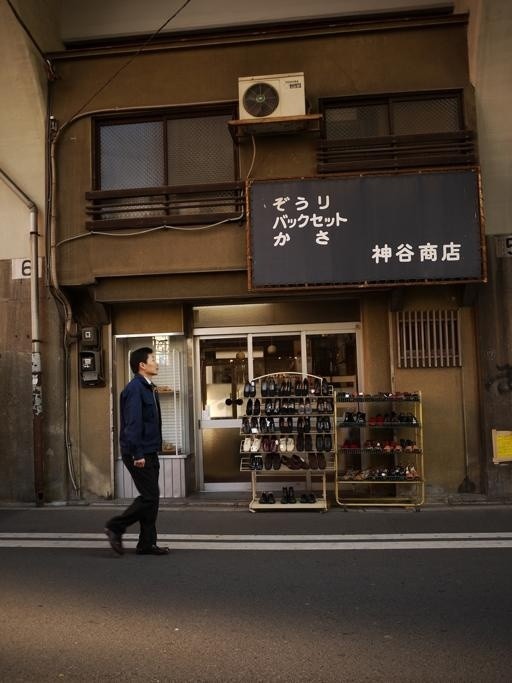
[239,371,335,513]
[128,348,180,456]
[331,388,425,511]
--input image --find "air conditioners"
[237,71,308,121]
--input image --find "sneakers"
[299,493,316,503]
[342,410,418,427]
[337,390,420,402]
[341,438,419,451]
[340,464,421,481]
[258,490,275,504]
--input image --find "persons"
[101,346,171,556]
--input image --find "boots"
[279,486,296,503]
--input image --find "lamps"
[267,336,277,354]
[236,338,245,360]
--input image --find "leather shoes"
[102,523,125,556]
[242,377,334,470]
[134,541,169,555]
